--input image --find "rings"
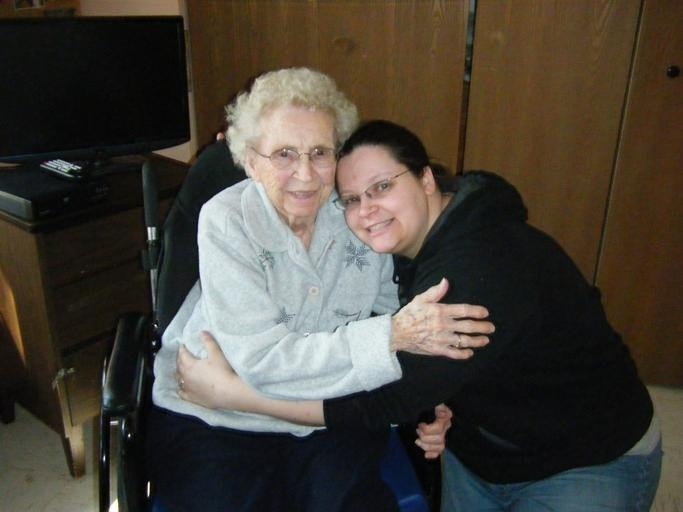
[178,378,185,390]
[456,333,462,349]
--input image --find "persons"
[170,118,667,511]
[134,68,497,510]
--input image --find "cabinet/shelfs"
[182,0,683,390]
[0,151,193,480]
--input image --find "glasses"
[247,144,339,167]
[332,167,413,213]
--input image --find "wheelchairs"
[100,140,443,512]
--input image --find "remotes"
[38,158,95,182]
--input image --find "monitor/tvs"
[0,15,191,170]
